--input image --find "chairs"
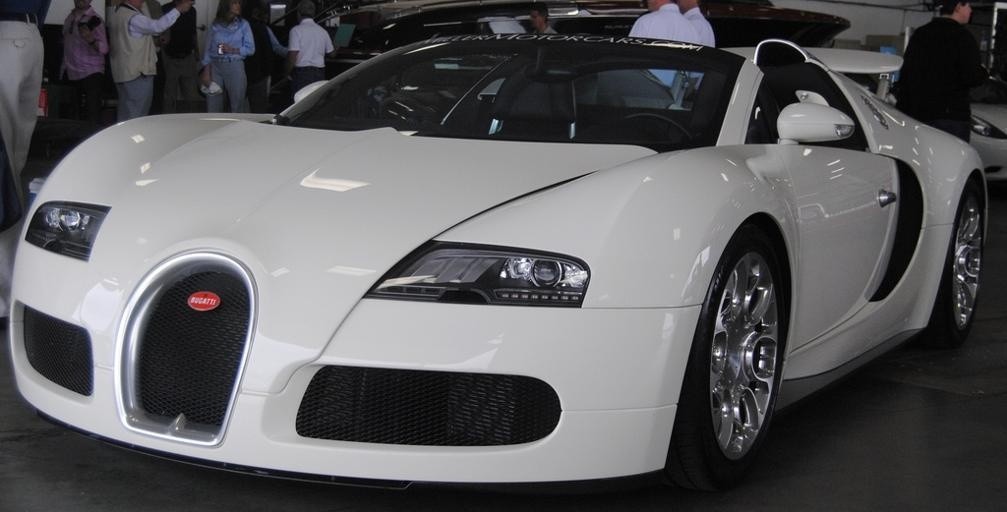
[487,65,577,141]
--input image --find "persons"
[58,0,107,129]
[202,1,333,113]
[675,1,718,109]
[525,0,557,38]
[108,1,200,123]
[894,1,991,143]
[0,1,51,188]
[629,1,702,107]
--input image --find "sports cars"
[8,2,1006,494]
[241,1,1007,194]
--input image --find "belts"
[212,57,243,63]
[1,11,36,23]
